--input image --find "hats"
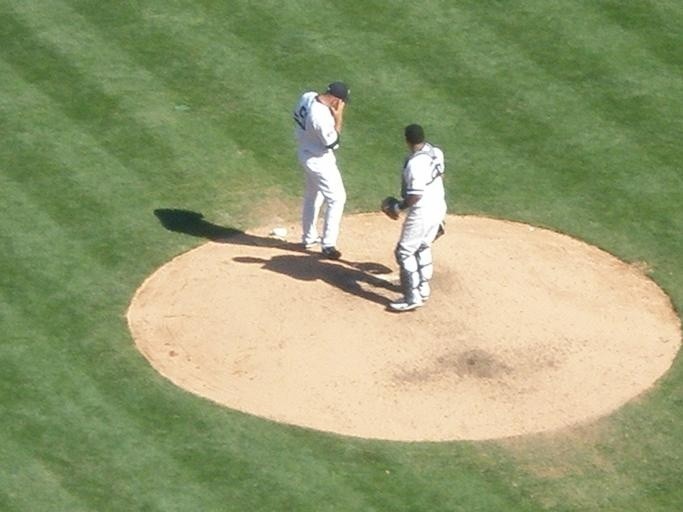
[403,121,427,144]
[327,79,351,105]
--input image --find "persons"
[290,81,350,261]
[378,123,449,312]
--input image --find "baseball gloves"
[381,197,399,220]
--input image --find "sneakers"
[387,296,423,312]
[301,235,324,248]
[320,245,343,261]
